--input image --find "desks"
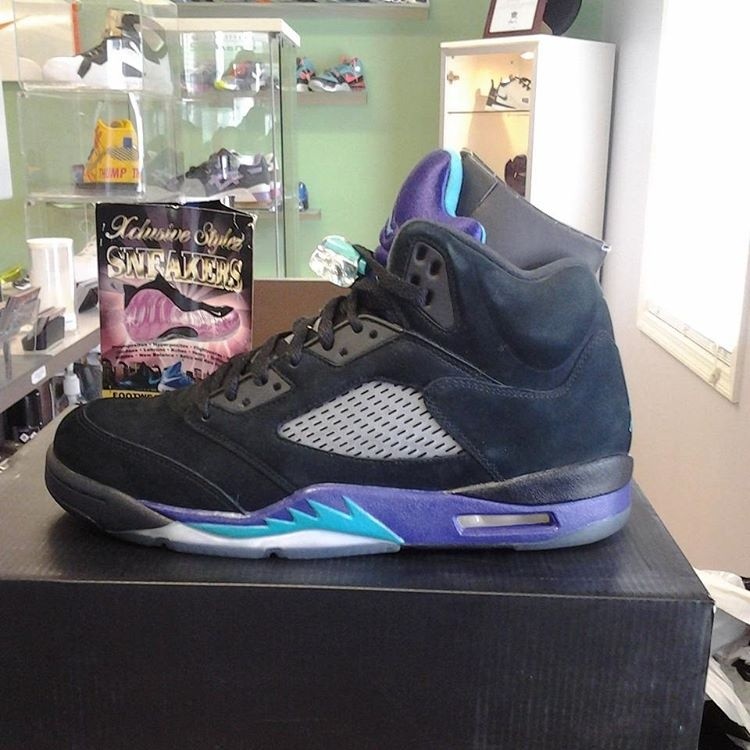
[0,305,102,429]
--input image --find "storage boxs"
[0,402,714,750]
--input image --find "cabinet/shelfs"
[175,0,431,222]
[17,1,301,318]
[438,34,616,284]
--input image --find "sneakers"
[504,154,527,196]
[144,136,185,191]
[157,361,195,393]
[75,118,141,195]
[276,57,315,92]
[179,59,216,91]
[166,147,279,204]
[120,271,240,345]
[118,361,163,392]
[215,60,266,92]
[43,146,634,561]
[308,55,366,94]
[484,74,533,112]
[42,14,173,94]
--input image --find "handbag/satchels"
[692,566,750,734]
